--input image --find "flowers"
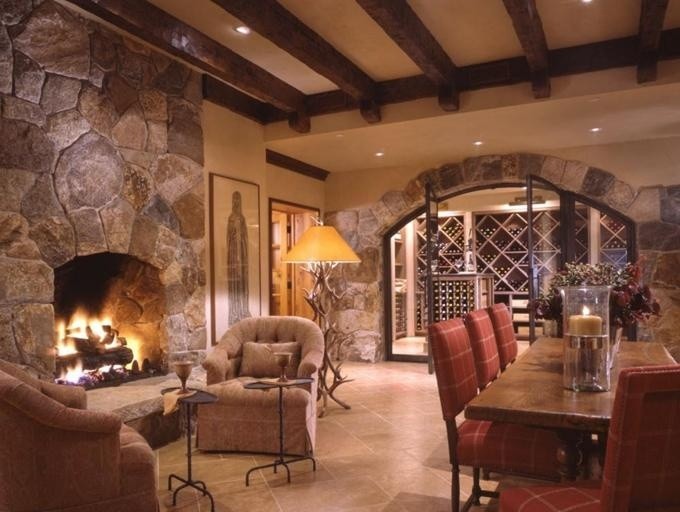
[526,258,662,322]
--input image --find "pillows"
[237,342,299,378]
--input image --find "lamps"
[282,222,363,420]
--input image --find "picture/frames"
[209,170,264,350]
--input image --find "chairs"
[196,313,330,465]
[0,355,180,510]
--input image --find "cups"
[556,284,613,392]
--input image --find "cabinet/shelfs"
[161,383,217,511]
[392,209,626,338]
[242,377,321,486]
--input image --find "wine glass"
[272,351,294,382]
[173,360,195,396]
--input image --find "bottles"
[395,280,473,332]
[417,213,627,291]
